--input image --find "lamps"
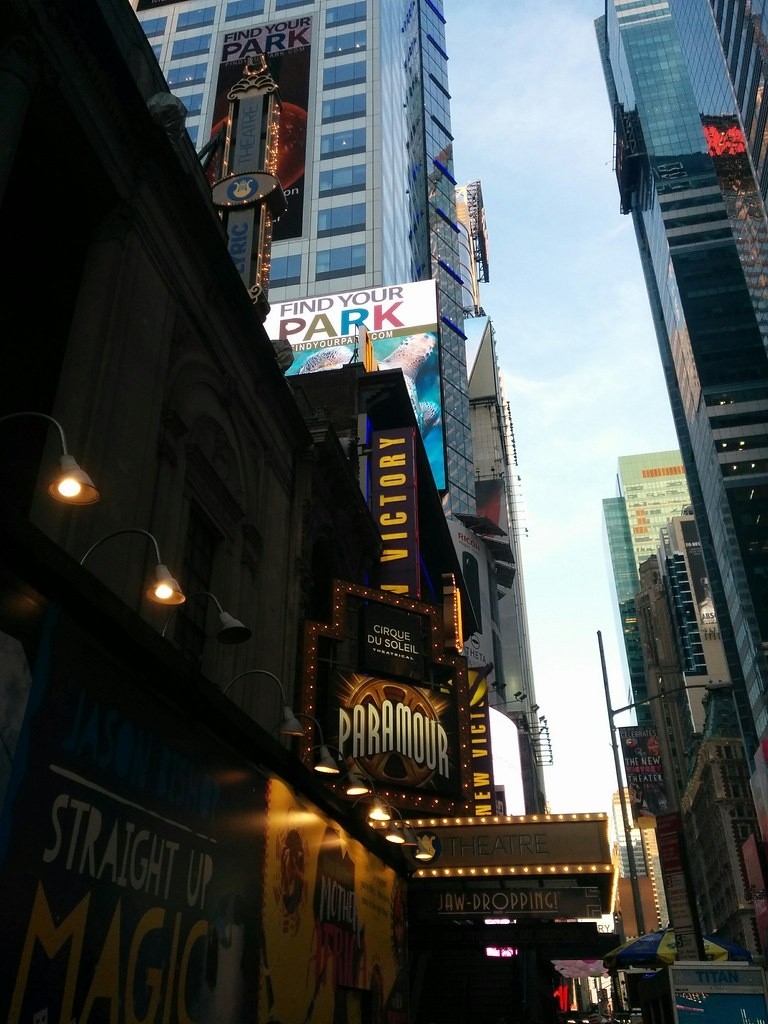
[272,714,341,773]
[303,743,431,858]
[223,668,307,737]
[83,526,185,605]
[161,591,252,642]
[0,408,102,505]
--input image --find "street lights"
[597,629,735,935]
